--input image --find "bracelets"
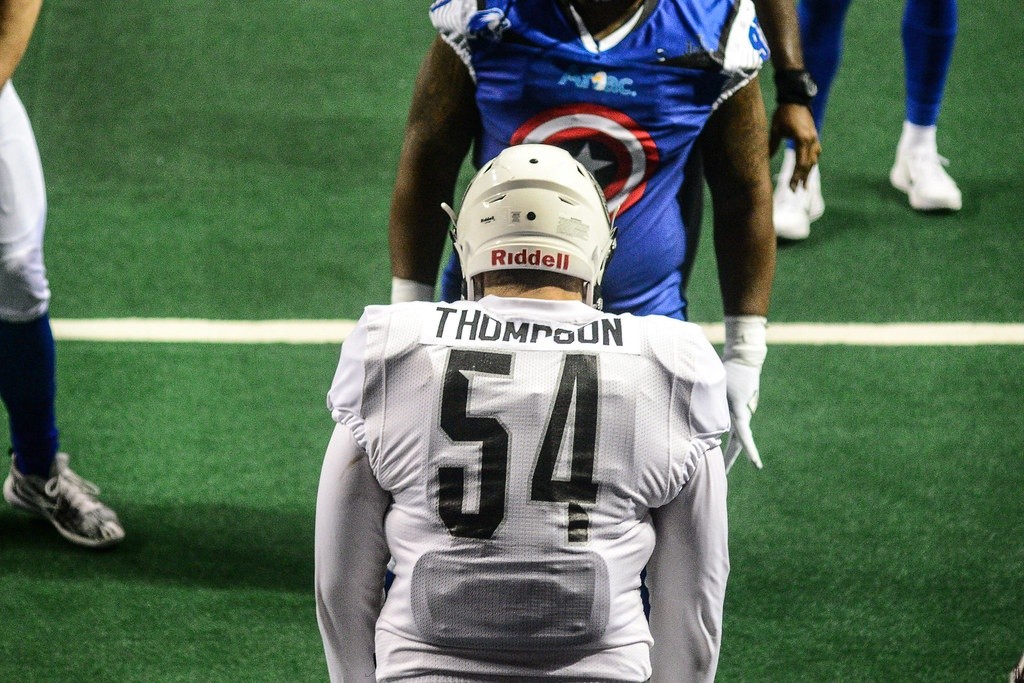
[773,69,818,105]
[391,277,433,305]
[723,316,769,367]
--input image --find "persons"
[314,144,730,683]
[388,0,777,477]
[0,0,125,548]
[752,0,963,243]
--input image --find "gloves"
[720,314,767,475]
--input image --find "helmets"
[439,142,620,309]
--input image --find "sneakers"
[770,145,828,242]
[1,446,126,548]
[889,121,963,215]
